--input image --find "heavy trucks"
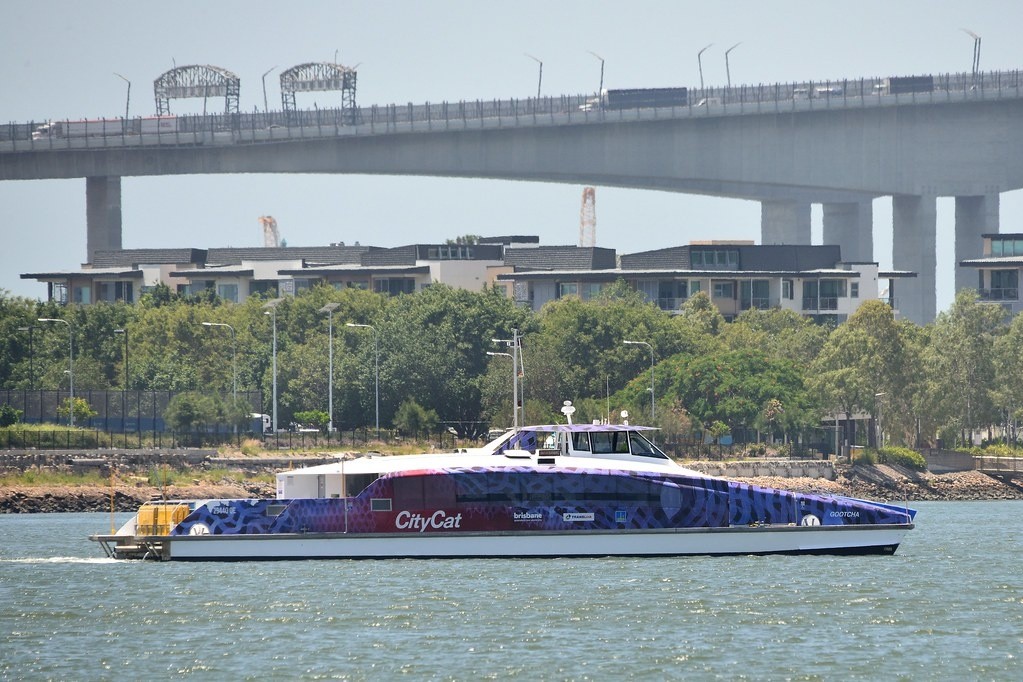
[29,116,181,141]
[579,87,687,113]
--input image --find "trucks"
[789,85,843,100]
[871,76,933,98]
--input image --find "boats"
[90,418,919,566]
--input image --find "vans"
[248,412,271,434]
[692,99,722,109]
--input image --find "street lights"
[586,49,604,112]
[723,41,743,104]
[37,316,74,427]
[624,339,656,445]
[28,324,42,393]
[521,52,543,107]
[115,328,130,433]
[262,64,281,130]
[347,322,379,431]
[112,70,131,136]
[263,303,279,437]
[962,27,981,93]
[315,302,340,433]
[875,393,886,450]
[202,319,236,435]
[487,338,522,429]
[698,42,711,106]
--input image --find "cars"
[274,421,337,435]
[483,429,505,445]
[266,123,289,130]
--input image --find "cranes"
[261,214,282,250]
[578,186,598,246]
